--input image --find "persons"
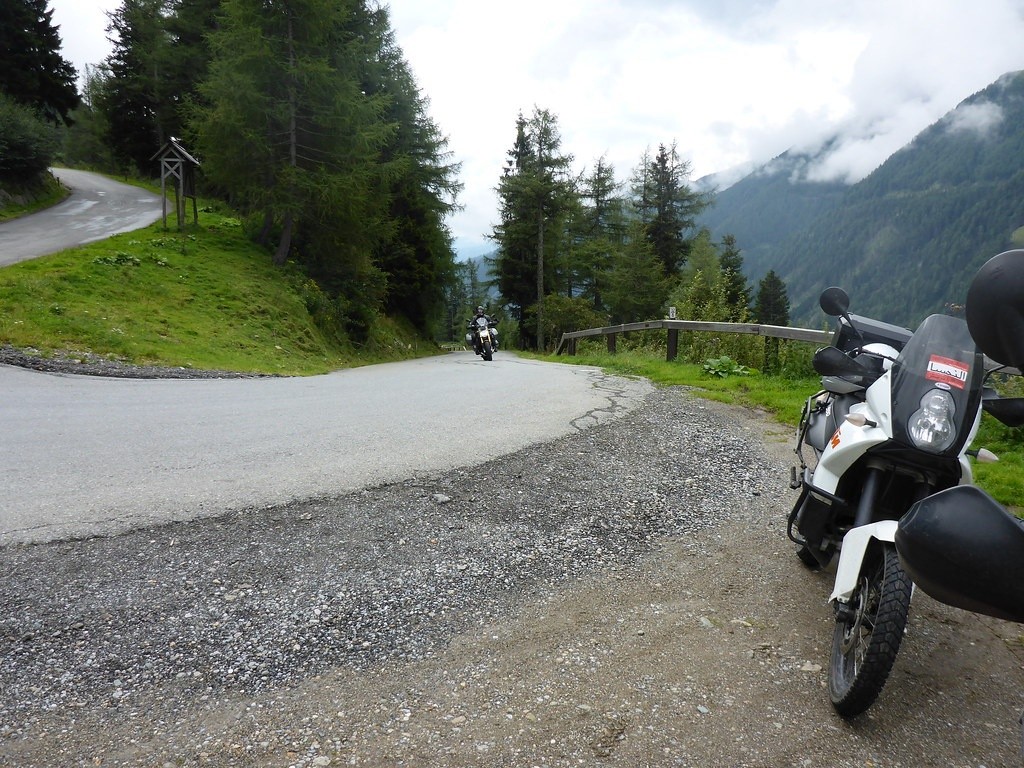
[468,306,499,354]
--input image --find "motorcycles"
[787,286,1023,716]
[465,313,499,361]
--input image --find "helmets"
[476,306,484,315]
